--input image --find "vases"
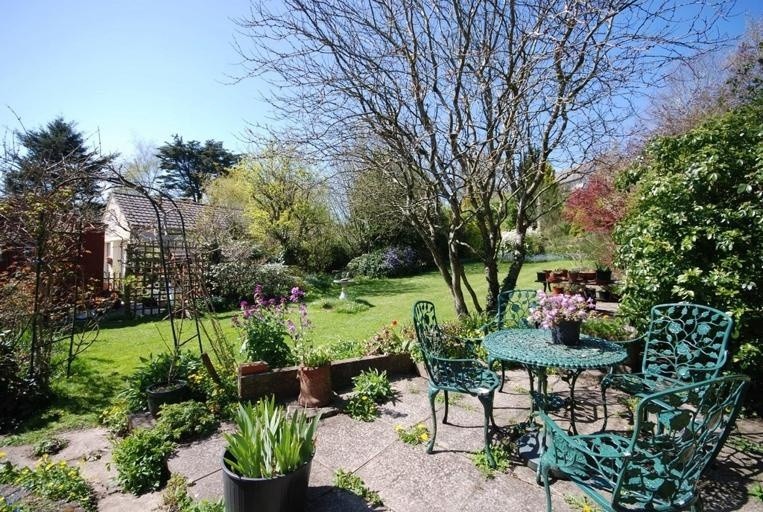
[547,315,580,344]
[536,268,596,283]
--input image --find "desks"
[535,279,622,293]
[482,324,629,440]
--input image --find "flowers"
[525,291,594,329]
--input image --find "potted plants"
[219,393,320,511]
[587,316,647,376]
[594,261,613,282]
[146,347,191,421]
[296,348,334,410]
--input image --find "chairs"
[496,288,553,416]
[413,299,501,470]
[539,371,753,512]
[594,303,734,442]
